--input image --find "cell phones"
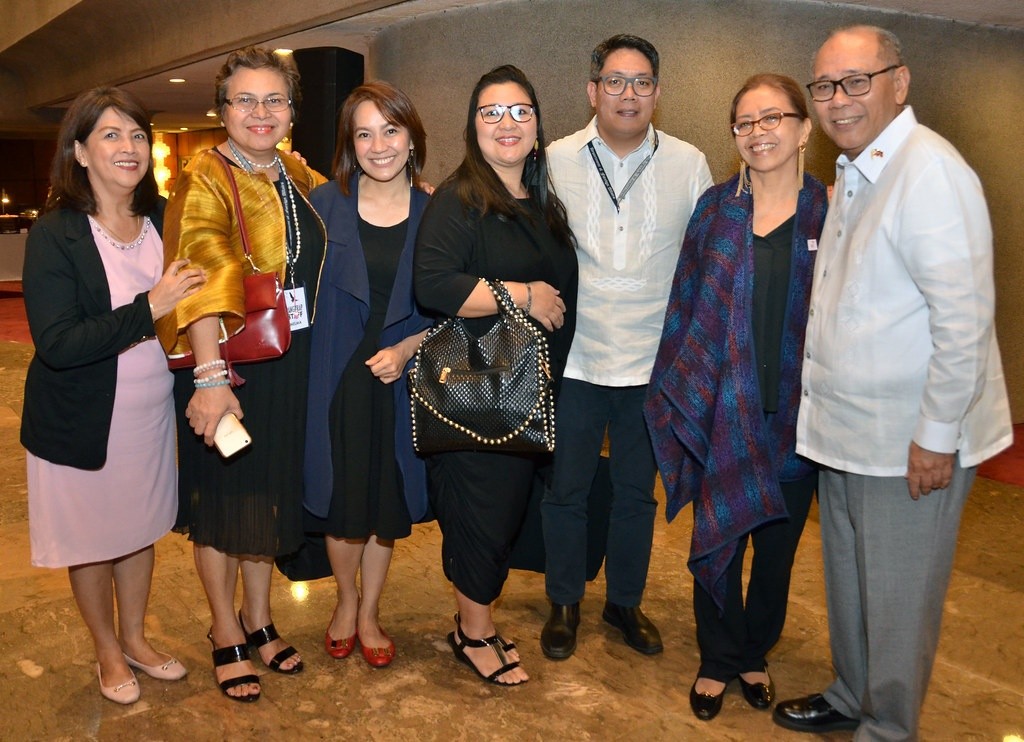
[212,412,251,457]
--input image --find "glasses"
[473,104,537,123]
[223,97,295,113]
[730,112,803,137]
[806,64,899,102]
[593,74,660,97]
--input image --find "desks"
[0,215,31,281]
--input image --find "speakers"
[289,46,365,182]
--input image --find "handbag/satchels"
[165,149,292,387]
[407,279,556,452]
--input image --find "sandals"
[454,611,521,664]
[207,626,262,702]
[239,609,304,673]
[448,612,527,685]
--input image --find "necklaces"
[228,137,301,266]
[97,214,140,243]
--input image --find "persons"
[529,34,714,661]
[20,85,304,703]
[773,23,1014,742]
[149,45,438,701]
[414,63,578,687]
[640,72,822,721]
[299,80,434,668]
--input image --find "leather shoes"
[324,594,360,658]
[541,603,580,657]
[357,621,397,667]
[96,657,140,704]
[602,600,664,654]
[689,677,728,720]
[123,650,188,681]
[771,692,862,731]
[737,666,775,709]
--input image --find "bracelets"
[192,359,231,387]
[149,303,155,325]
[524,283,532,317]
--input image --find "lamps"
[2,189,10,213]
